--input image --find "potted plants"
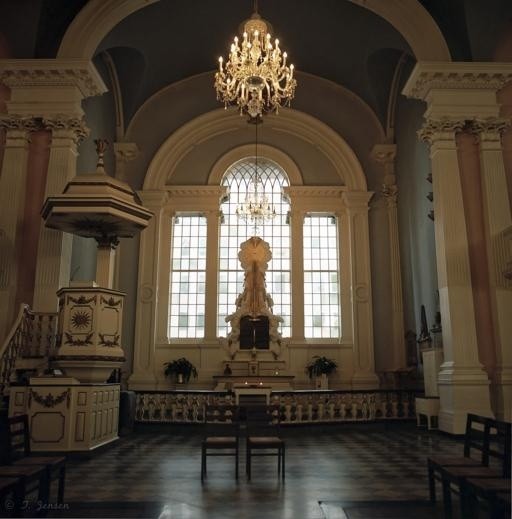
[305,354,338,390]
[162,356,199,385]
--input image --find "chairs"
[200,404,286,485]
[0,414,69,519]
[426,413,512,519]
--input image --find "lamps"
[236,127,277,230]
[210,0,298,126]
